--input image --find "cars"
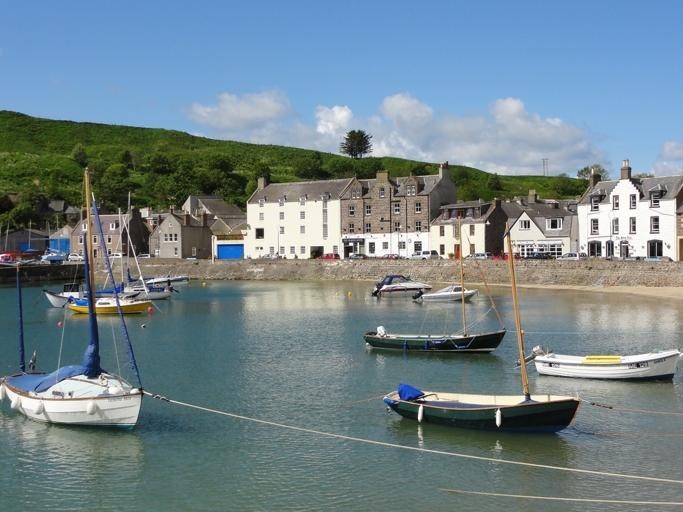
[349,253,369,260]
[376,253,404,260]
[314,252,340,260]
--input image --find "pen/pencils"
[555,251,587,261]
[405,250,439,261]
[463,251,495,260]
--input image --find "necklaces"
[362,214,507,356]
[381,222,584,437]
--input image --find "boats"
[368,275,434,300]
[514,342,683,380]
[418,281,480,303]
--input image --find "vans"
[555,251,587,261]
[405,250,439,261]
[463,251,495,260]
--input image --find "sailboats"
[2,163,144,429]
[381,222,584,437]
[41,191,181,315]
[362,214,507,356]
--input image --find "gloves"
[418,281,480,303]
[368,275,434,300]
[514,342,683,380]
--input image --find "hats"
[349,253,369,260]
[314,252,340,260]
[376,253,404,260]
